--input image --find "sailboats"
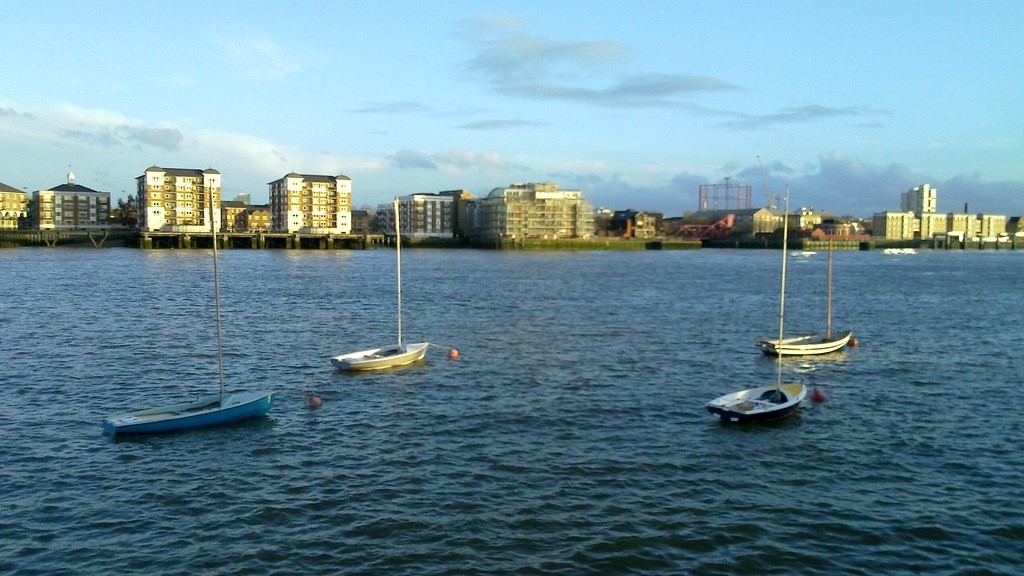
[705,183,807,423]
[754,238,853,357]
[102,177,277,437]
[329,196,429,372]
